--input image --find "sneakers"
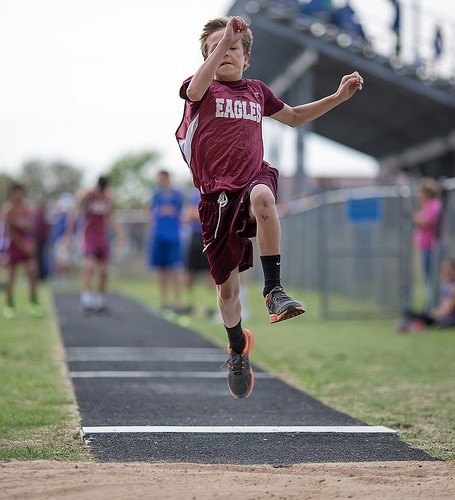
[227,328,254,401]
[264,285,305,325]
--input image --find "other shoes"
[4,303,17,318]
[204,309,217,320]
[27,303,46,318]
[159,304,180,317]
[398,318,424,334]
[181,307,195,317]
[83,306,110,317]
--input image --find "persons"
[0,165,243,316]
[174,14,364,400]
[400,172,454,334]
